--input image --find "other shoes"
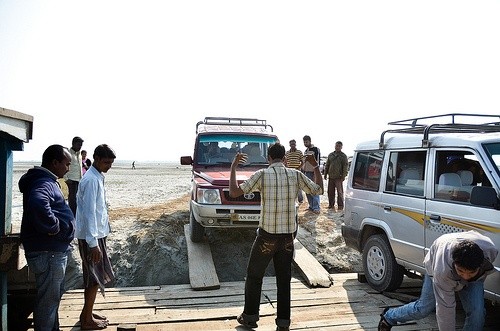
[237,314,258,328]
[378,306,393,331]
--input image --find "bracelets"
[312,165,319,169]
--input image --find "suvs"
[179,116,287,243]
[340,112,500,307]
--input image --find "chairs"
[396,169,420,196]
[438,173,462,200]
[244,146,265,164]
[459,171,473,193]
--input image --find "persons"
[18,144,75,331]
[303,135,321,214]
[132,161,135,169]
[75,144,116,330]
[63,136,84,216]
[207,143,220,154]
[229,142,324,331]
[81,150,91,175]
[285,139,304,203]
[378,230,498,331]
[324,141,348,210]
